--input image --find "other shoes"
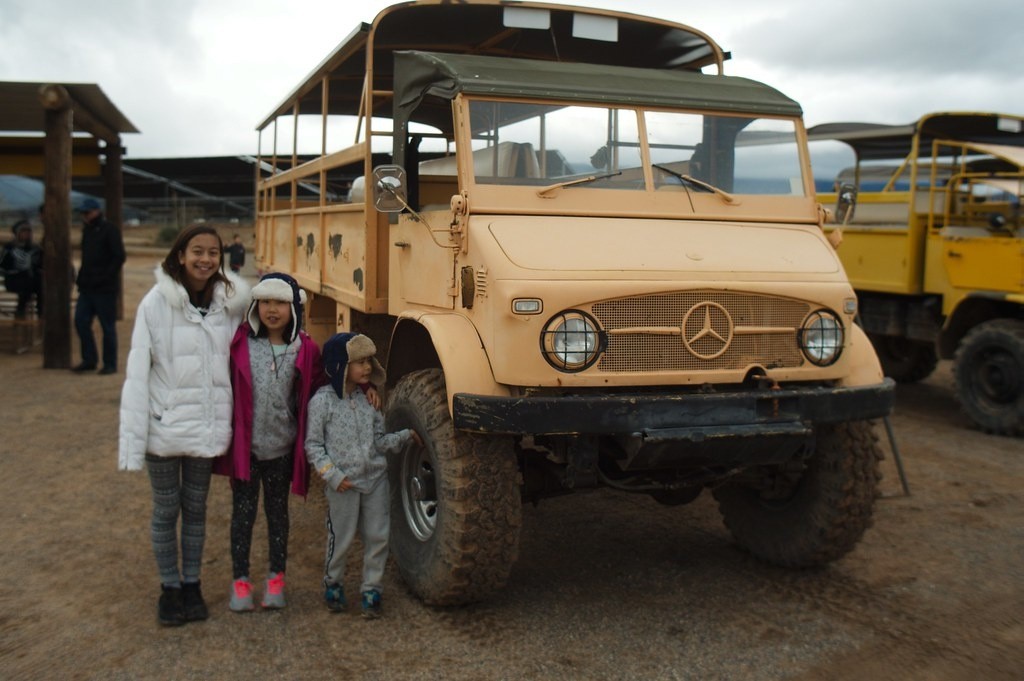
[158,583,185,624]
[73,359,100,373]
[97,363,117,375]
[182,578,210,621]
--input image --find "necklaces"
[268,337,289,380]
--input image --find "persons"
[118,222,251,625]
[213,272,382,612]
[304,331,424,619]
[0,220,44,321]
[74,200,127,374]
[30,202,76,296]
[224,233,246,272]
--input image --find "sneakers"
[262,571,286,608]
[229,575,254,611]
[324,583,347,612]
[358,591,382,619]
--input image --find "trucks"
[726,108,1024,441]
[251,0,900,611]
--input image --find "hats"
[69,198,101,214]
[321,332,388,401]
[247,272,303,342]
